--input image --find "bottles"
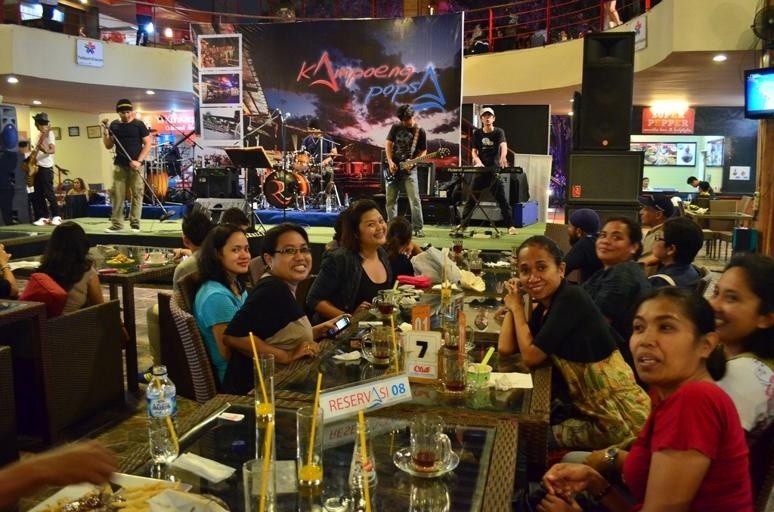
[348,419,378,511]
[326,196,332,214]
[146,363,175,463]
[242,350,324,511]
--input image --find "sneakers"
[105,226,123,233]
[32,216,49,226]
[129,224,139,233]
[411,229,425,237]
[507,226,516,235]
[51,216,61,225]
[137,366,153,384]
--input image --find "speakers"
[0,152,32,225]
[578,30,638,154]
[566,209,640,228]
[0,105,20,152]
[568,151,646,207]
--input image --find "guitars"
[383,147,449,183]
[23,128,53,186]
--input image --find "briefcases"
[512,200,538,228]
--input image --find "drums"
[293,151,313,171]
[149,171,169,200]
[264,169,310,208]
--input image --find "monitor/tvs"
[743,66,774,118]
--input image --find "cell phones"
[327,316,353,338]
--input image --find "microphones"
[282,112,291,125]
[248,125,272,138]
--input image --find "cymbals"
[323,152,343,156]
[303,128,324,133]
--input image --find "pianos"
[447,167,522,175]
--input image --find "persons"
[565,254,774,463]
[307,200,421,317]
[565,191,705,320]
[68,177,89,200]
[464,3,619,55]
[173,208,262,287]
[642,178,653,191]
[497,235,653,448]
[687,176,713,194]
[19,112,61,225]
[136,0,152,45]
[383,105,426,237]
[0,436,117,510]
[192,222,250,382]
[103,99,152,233]
[1,243,19,296]
[302,119,337,209]
[220,225,354,395]
[39,221,104,314]
[541,285,754,510]
[454,108,515,230]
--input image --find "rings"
[304,344,311,349]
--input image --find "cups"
[147,252,162,263]
[411,482,450,511]
[443,320,474,354]
[360,326,394,365]
[408,414,451,472]
[467,363,492,387]
[372,290,399,317]
[325,496,346,511]
[452,240,483,277]
[439,356,476,394]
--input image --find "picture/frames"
[629,141,697,167]
[68,126,79,137]
[86,125,101,139]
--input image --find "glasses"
[273,246,311,255]
[653,233,665,241]
[467,297,503,308]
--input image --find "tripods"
[243,209,268,234]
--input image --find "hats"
[637,192,673,218]
[479,107,495,116]
[568,208,601,236]
[32,112,50,122]
[116,99,132,113]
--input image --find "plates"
[143,261,168,267]
[430,378,480,396]
[391,447,460,477]
[29,472,192,511]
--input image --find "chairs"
[678,194,756,261]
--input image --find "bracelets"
[592,485,614,501]
[2,265,10,270]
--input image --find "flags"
[74,39,103,68]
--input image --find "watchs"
[603,446,621,469]
